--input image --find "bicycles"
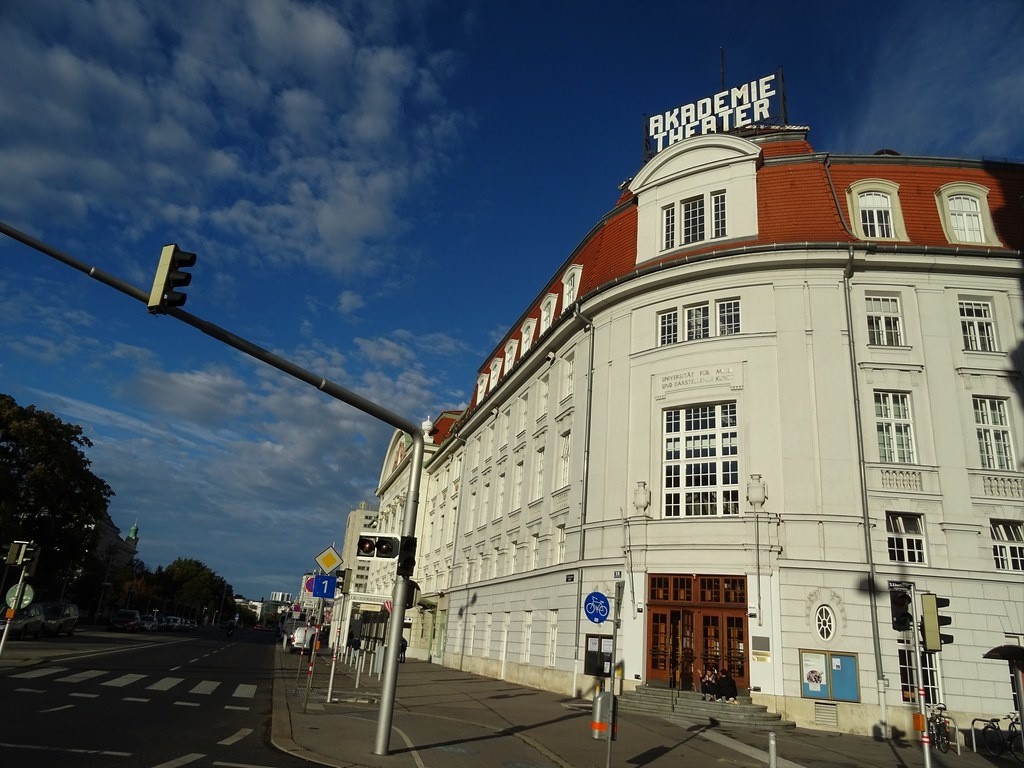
[982,712,1024,764]
[926,703,950,753]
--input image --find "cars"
[0,601,79,640]
[140,615,158,632]
[156,616,198,633]
[107,609,142,633]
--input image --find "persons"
[307,634,315,663]
[347,630,361,664]
[700,667,718,702]
[399,636,407,663]
[714,669,738,703]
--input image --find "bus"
[276,611,307,641]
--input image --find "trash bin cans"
[591,691,619,741]
[373,646,388,673]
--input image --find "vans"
[289,626,316,654]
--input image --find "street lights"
[397,537,417,576]
[211,610,219,631]
[356,536,397,557]
[93,582,112,625]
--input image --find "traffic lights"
[921,593,953,651]
[889,590,914,630]
[18,543,37,566]
[146,243,196,316]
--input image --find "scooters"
[227,624,235,638]
